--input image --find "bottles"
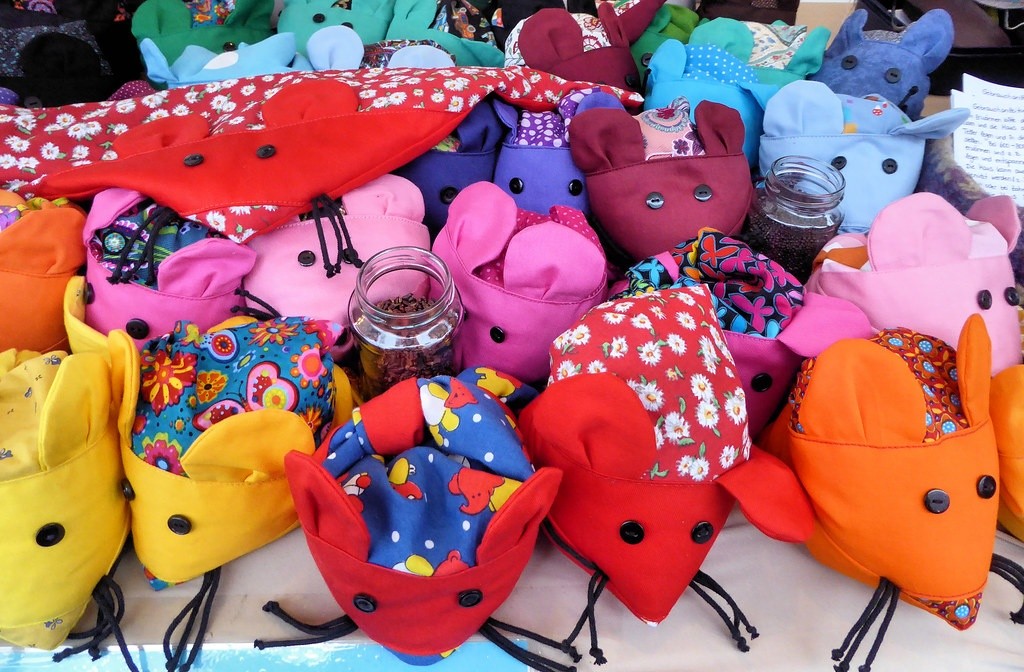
[347,246,465,403]
[744,155,847,278]
